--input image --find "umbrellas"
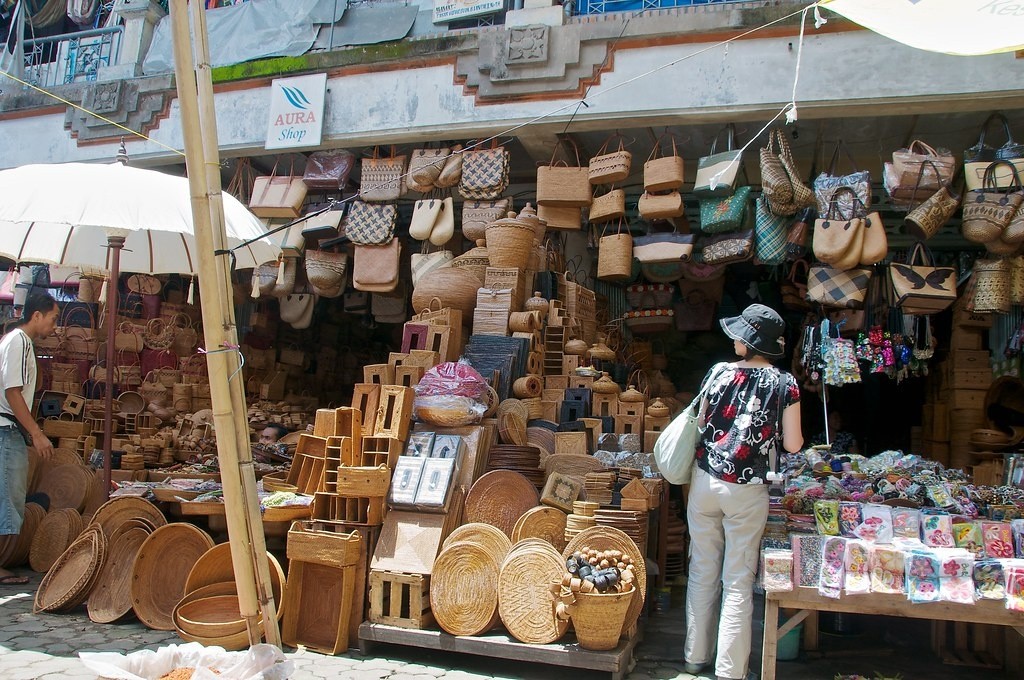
[0,135,284,503]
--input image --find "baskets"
[29,264,400,651]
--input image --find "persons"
[807,407,858,454]
[0,291,60,584]
[684,303,805,680]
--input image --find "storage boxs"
[267,324,430,658]
[540,470,582,514]
[513,298,669,456]
[911,306,993,471]
[33,389,155,464]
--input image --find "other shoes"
[718,667,759,680]
[685,660,711,674]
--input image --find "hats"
[720,304,785,358]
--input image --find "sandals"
[0,568,30,585]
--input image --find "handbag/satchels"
[225,111,1024,432]
[0,412,32,447]
[653,362,727,486]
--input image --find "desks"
[760,585,1024,680]
